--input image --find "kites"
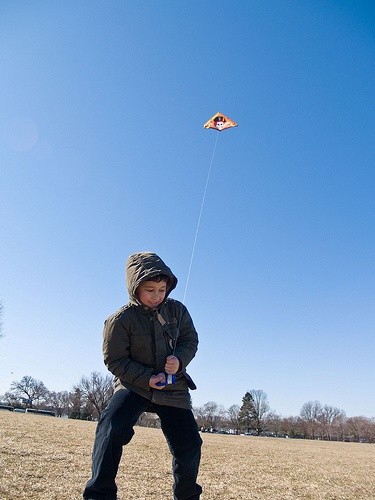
[203,112,239,132]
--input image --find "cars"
[198,426,305,439]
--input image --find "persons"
[81,252,204,500]
[198,423,329,441]
